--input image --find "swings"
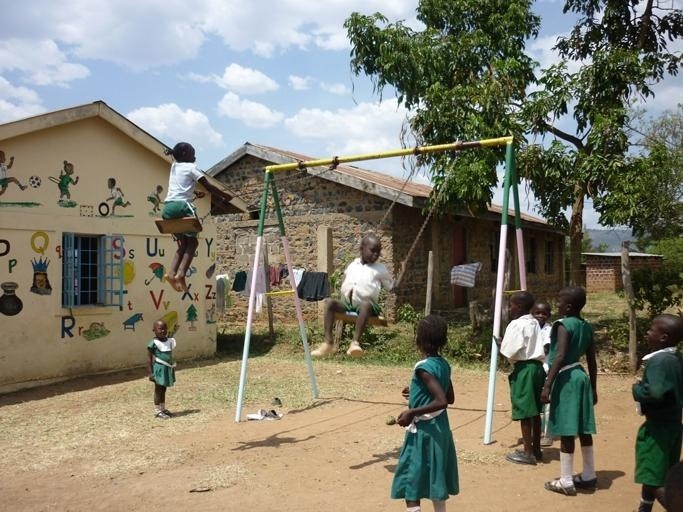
[153,157,339,233]
[324,142,458,325]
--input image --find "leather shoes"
[505,447,543,464]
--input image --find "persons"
[161,141,234,294]
[0,150,28,196]
[530,301,553,446]
[631,314,683,512]
[493,291,547,466]
[146,318,177,420]
[310,234,407,359]
[664,459,683,512]
[539,285,599,497]
[58,160,79,202]
[105,178,131,216]
[390,313,460,512]
[147,184,164,213]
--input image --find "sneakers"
[346,341,363,357]
[311,343,336,357]
[266,410,280,419]
[545,473,598,495]
[271,397,282,406]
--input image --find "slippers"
[156,410,174,419]
